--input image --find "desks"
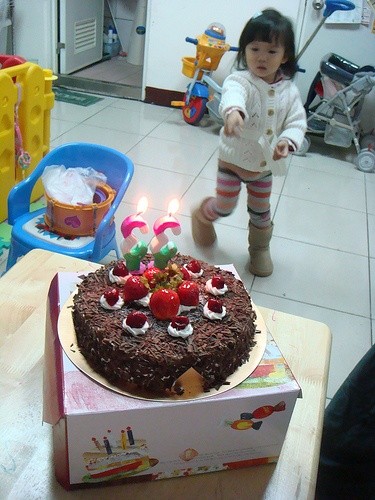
[0,247,331,500]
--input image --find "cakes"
[71,251,258,399]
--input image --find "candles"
[119,196,150,271]
[150,199,181,269]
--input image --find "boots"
[248,219,274,276]
[191,196,217,247]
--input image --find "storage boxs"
[47,264,302,491]
[103,41,120,57]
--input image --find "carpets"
[55,89,101,106]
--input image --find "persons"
[190,9,307,277]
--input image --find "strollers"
[292,51,375,173]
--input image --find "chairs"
[5,143,134,271]
